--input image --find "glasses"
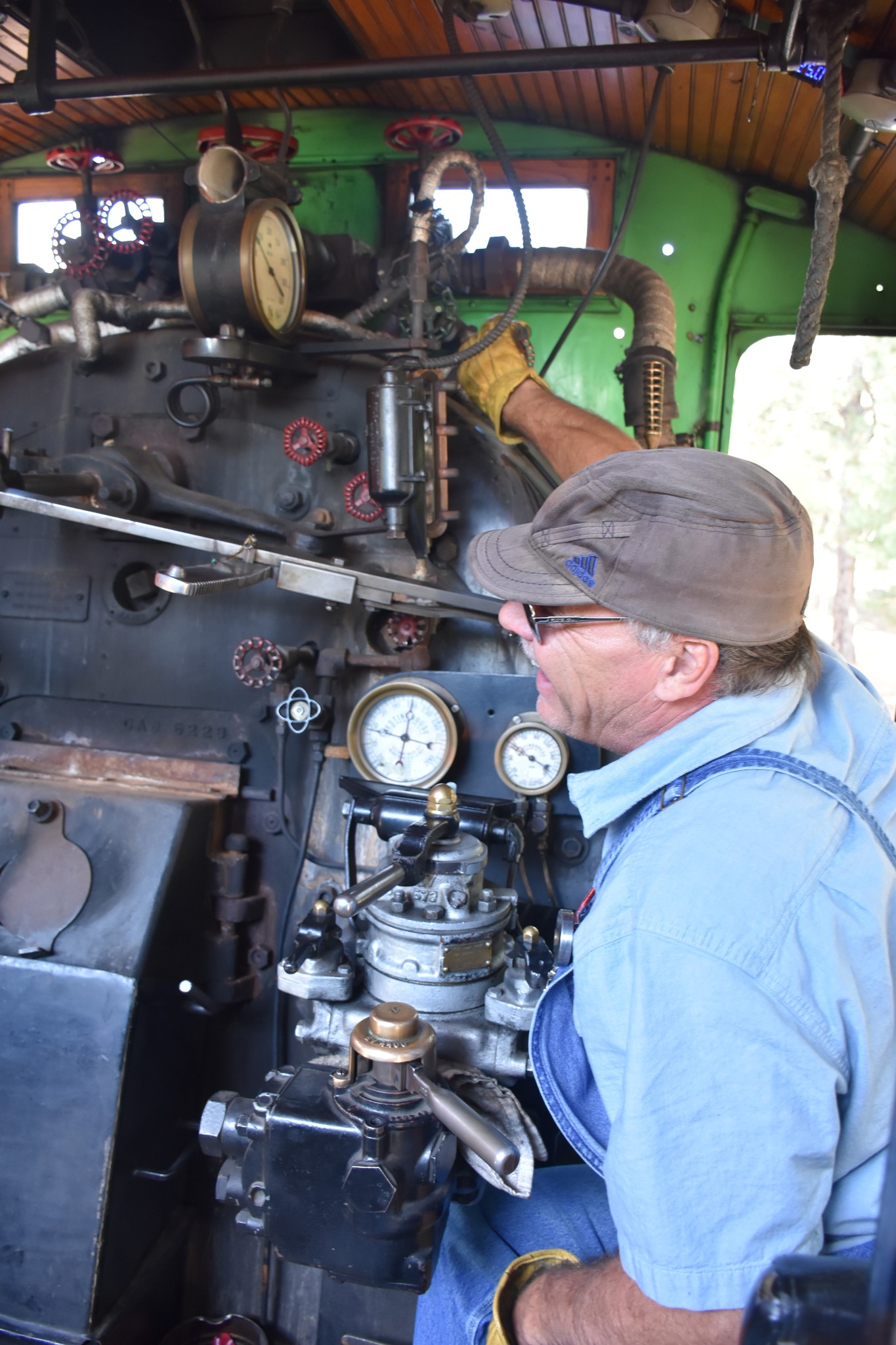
[523,600,632,644]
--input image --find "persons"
[413,317,896,1345]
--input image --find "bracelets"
[509,1263,552,1345]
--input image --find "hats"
[465,448,813,647]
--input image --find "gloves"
[488,1248,580,1345]
[457,315,551,444]
